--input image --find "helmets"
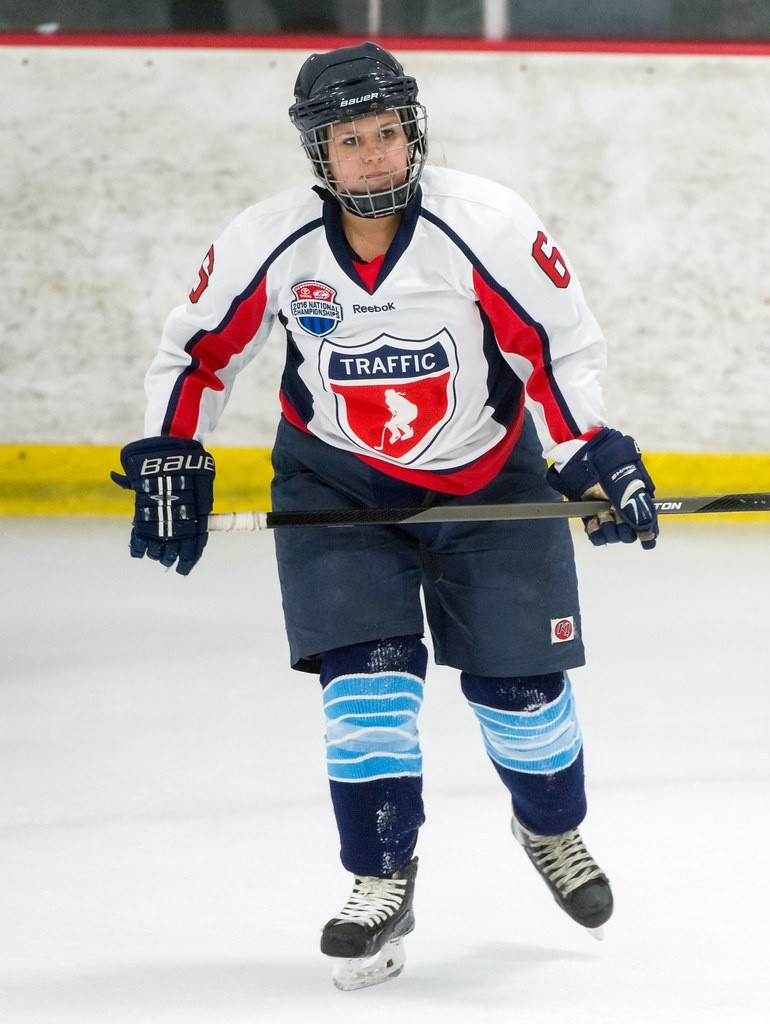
[290,41,427,219]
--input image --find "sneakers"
[320,856,419,992]
[513,811,614,941]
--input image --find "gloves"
[546,428,659,550]
[109,437,216,576]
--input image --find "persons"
[110,41,659,993]
